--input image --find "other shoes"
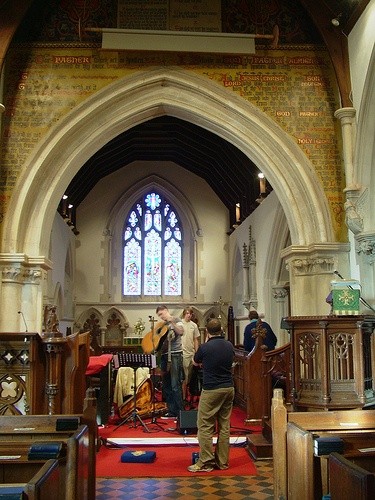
[161,412,177,420]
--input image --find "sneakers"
[187,464,214,473]
[214,460,229,470]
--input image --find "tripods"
[112,353,168,433]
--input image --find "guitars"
[141,305,182,354]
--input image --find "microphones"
[334,271,375,313]
[18,311,28,332]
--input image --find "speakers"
[176,410,198,435]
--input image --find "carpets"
[96,395,264,476]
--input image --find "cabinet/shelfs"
[284,314,375,413]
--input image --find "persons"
[155,304,185,420]
[181,307,200,405]
[243,310,277,353]
[188,319,236,472]
